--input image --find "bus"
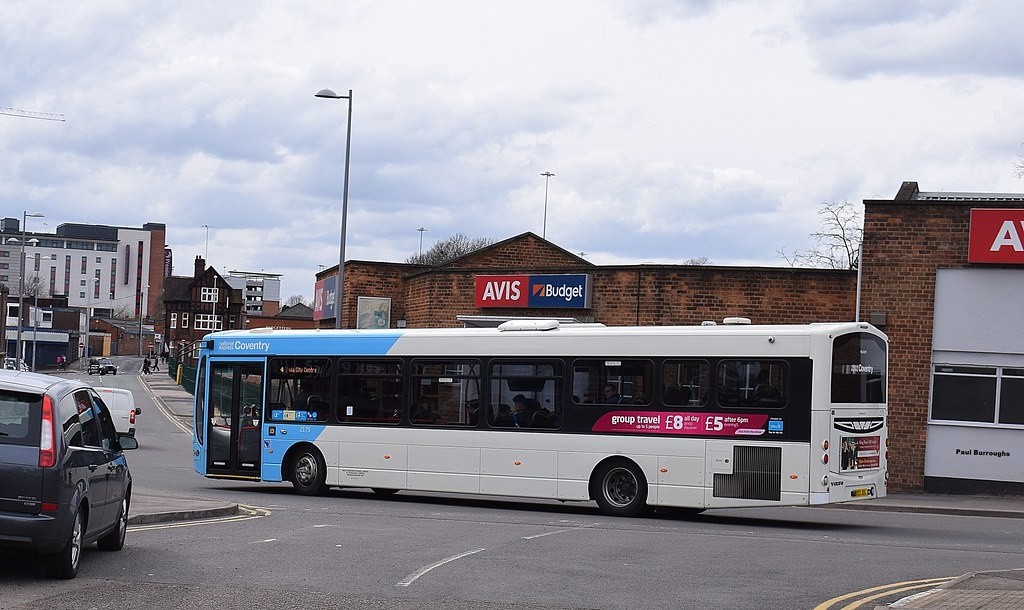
[171,316,890,520]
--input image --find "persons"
[143,355,152,375]
[62,354,67,371]
[841,441,858,471]
[510,394,530,428]
[147,349,157,359]
[57,354,62,371]
[153,356,159,371]
[466,399,480,424]
[160,350,166,364]
[604,383,625,404]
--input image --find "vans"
[90,385,142,443]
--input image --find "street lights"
[315,87,354,328]
[16,211,45,372]
[541,171,554,239]
[201,223,214,262]
[25,254,50,374]
[417,226,429,263]
[8,237,39,367]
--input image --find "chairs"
[514,411,529,427]
[662,385,691,406]
[754,384,780,402]
[532,411,547,428]
[618,398,629,405]
[546,413,559,428]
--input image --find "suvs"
[87,357,118,376]
[1,367,139,582]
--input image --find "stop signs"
[148,343,153,349]
[79,342,83,348]
